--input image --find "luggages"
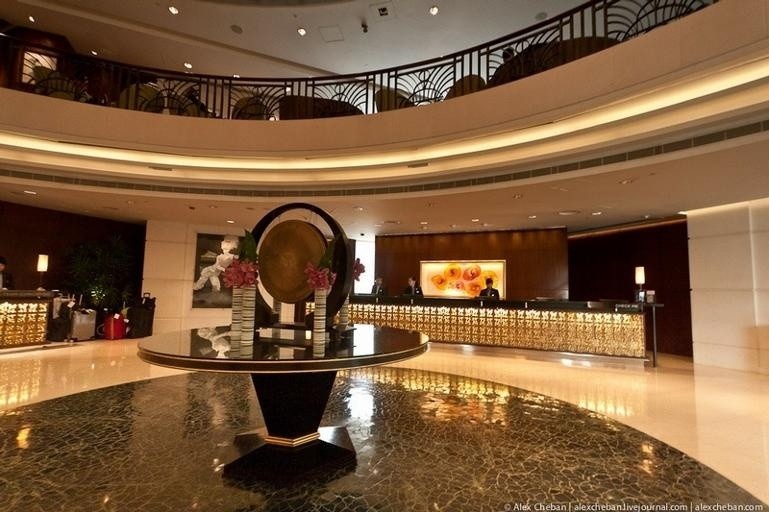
[47,317,70,342]
[128,293,156,339]
[69,308,97,343]
[105,313,126,340]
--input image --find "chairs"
[29,61,266,120]
[372,74,484,115]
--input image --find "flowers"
[305,260,335,290]
[222,258,261,286]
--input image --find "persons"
[371,277,387,295]
[480,278,499,300]
[0,256,15,291]
[405,276,424,298]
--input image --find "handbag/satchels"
[131,295,155,311]
[57,302,81,320]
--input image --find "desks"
[138,318,431,485]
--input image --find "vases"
[312,289,326,358]
[230,285,257,356]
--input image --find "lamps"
[36,253,49,291]
[635,266,646,304]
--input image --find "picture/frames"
[418,259,507,304]
